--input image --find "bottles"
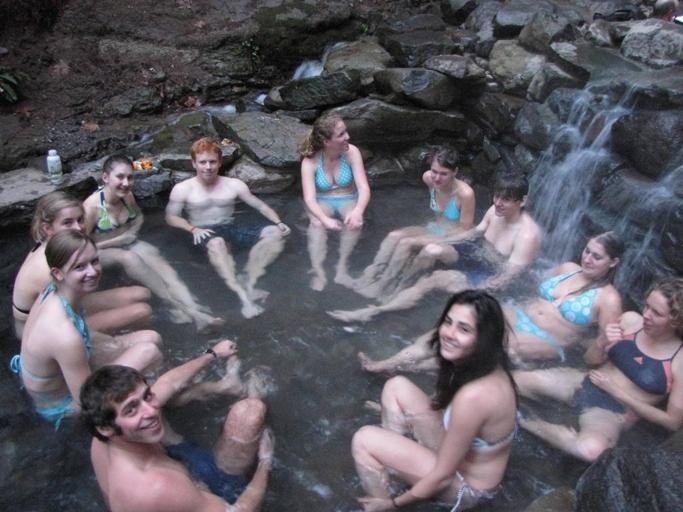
[45,149,64,186]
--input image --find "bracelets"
[190,226,195,234]
[391,497,399,509]
[276,221,283,225]
[204,348,216,359]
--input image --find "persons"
[20,229,164,426]
[301,114,371,292]
[163,137,290,320]
[79,339,275,512]
[358,230,622,373]
[344,144,475,300]
[325,168,541,323]
[13,191,152,342]
[82,155,226,332]
[351,288,519,512]
[509,276,683,462]
